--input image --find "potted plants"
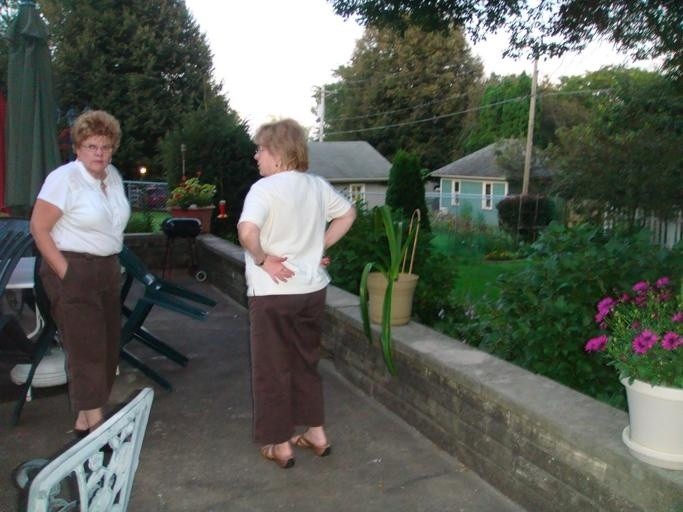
[358,205,420,377]
[166,177,217,234]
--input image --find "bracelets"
[255,254,268,266]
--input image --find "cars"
[133,183,168,211]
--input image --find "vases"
[619,373,682,468]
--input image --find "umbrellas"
[0,1,62,221]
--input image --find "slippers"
[261,433,331,468]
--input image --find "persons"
[28,108,131,454]
[235,116,358,470]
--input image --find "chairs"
[29,382,153,512]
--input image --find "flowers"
[583,273,682,391]
